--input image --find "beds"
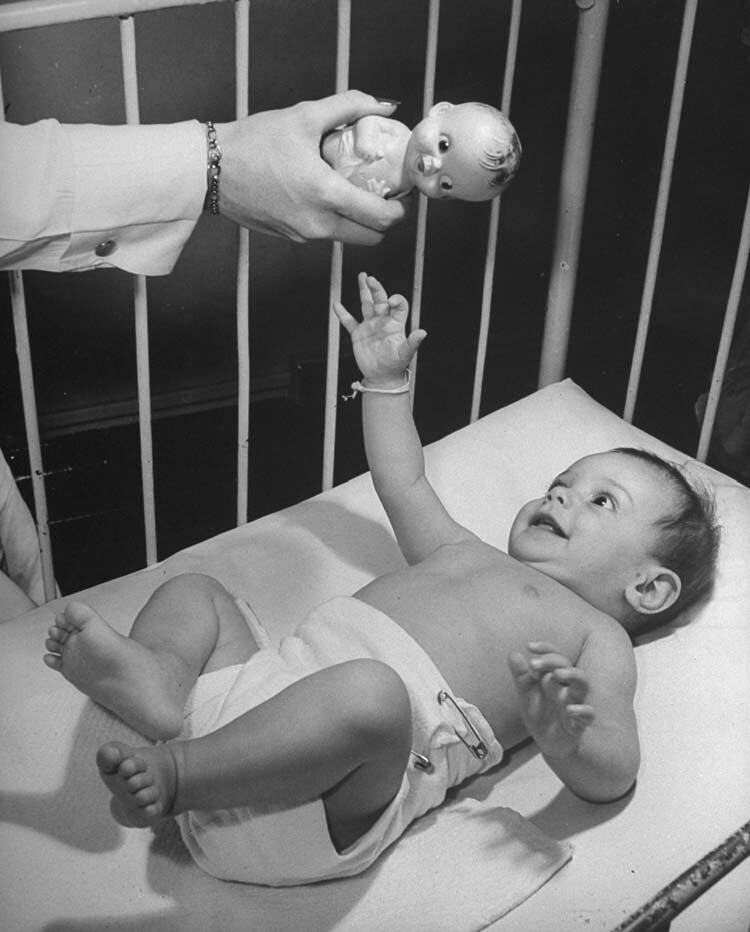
[1,3,749,932]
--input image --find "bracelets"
[340,366,413,402]
[204,120,222,216]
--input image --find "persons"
[43,270,724,889]
[321,100,526,211]
[0,85,415,630]
